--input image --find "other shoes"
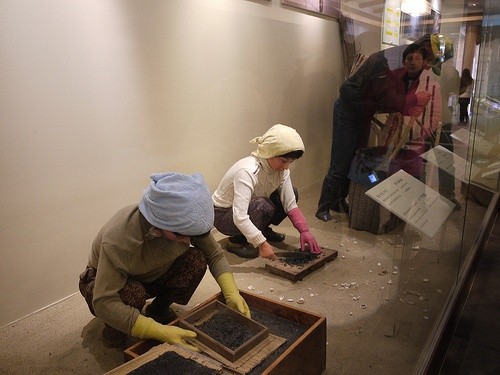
[331,197,349,212]
[144,303,179,325]
[263,227,285,241]
[102,324,126,348]
[227,241,259,258]
[316,209,331,222]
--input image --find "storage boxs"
[125,289,327,375]
[179,299,269,363]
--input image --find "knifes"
[273,250,311,259]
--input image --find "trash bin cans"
[348,146,422,235]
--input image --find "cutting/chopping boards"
[264,244,338,281]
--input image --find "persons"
[459,68,474,126]
[79,173,252,353]
[212,125,323,260]
[316,32,452,223]
[384,45,461,233]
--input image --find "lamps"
[400,0,432,17]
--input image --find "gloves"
[130,313,200,352]
[287,207,323,254]
[218,272,251,319]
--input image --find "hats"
[425,32,454,76]
